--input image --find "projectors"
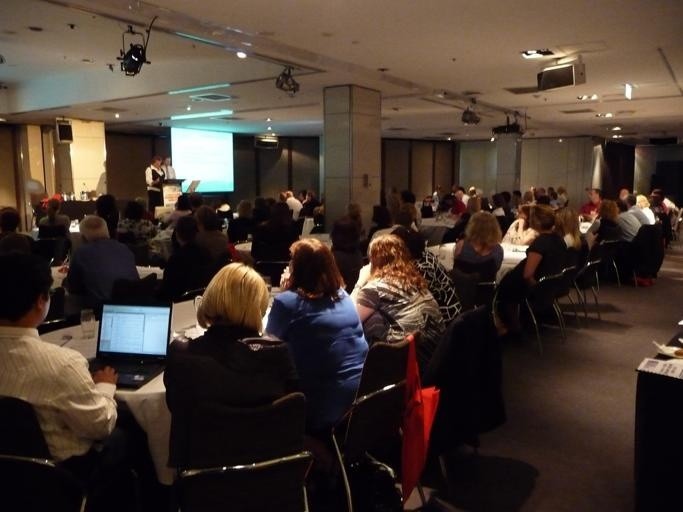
[492,120,524,136]
[252,133,280,149]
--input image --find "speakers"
[53,116,74,145]
[535,64,585,91]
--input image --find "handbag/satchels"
[402,386,442,500]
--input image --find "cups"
[61,191,75,202]
[79,308,95,340]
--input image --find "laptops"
[89,303,174,388]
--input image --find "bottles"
[79,182,89,201]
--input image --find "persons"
[1,184,678,371]
[160,156,177,181]
[1,231,122,505]
[144,156,165,213]
[264,238,395,489]
[160,262,304,472]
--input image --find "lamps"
[117,22,151,77]
[462,106,481,125]
[276,64,299,94]
